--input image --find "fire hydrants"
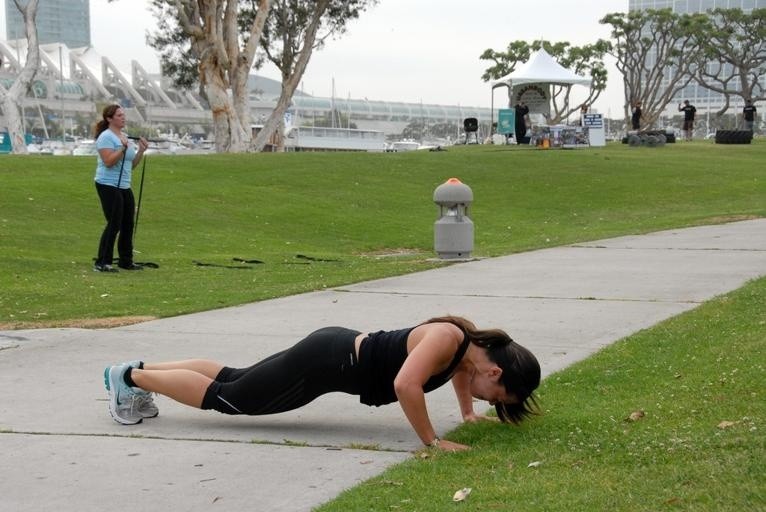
[432,177,475,261]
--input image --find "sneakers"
[103,364,143,424]
[92,262,118,273]
[121,360,159,418]
[118,261,143,270]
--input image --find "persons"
[632,101,646,131]
[679,100,698,142]
[92,103,148,273]
[743,99,758,140]
[103,314,543,452]
[514,102,529,145]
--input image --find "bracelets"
[425,433,440,447]
